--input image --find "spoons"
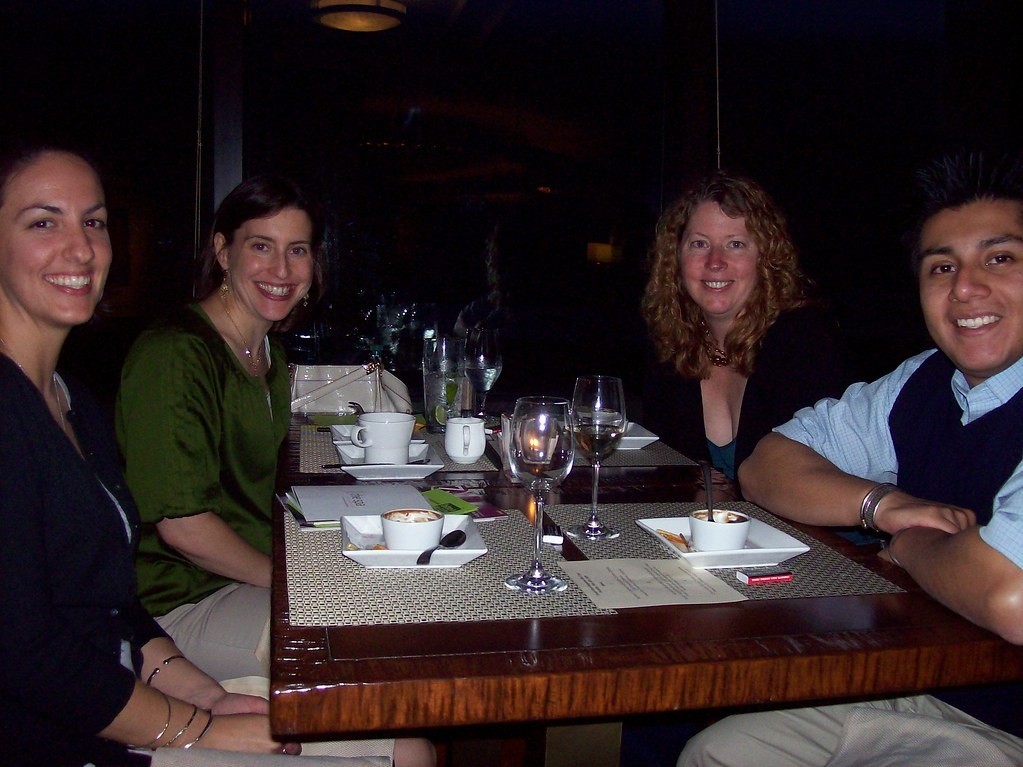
[321,459,430,469]
[415,530,466,565]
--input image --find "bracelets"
[145,655,186,686]
[142,692,214,750]
[861,483,899,532]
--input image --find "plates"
[635,513,812,569]
[330,424,365,442]
[611,421,660,449]
[333,441,446,479]
[340,514,488,569]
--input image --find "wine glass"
[463,327,502,426]
[572,373,622,540]
[505,397,576,592]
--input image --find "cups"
[444,416,486,464]
[349,412,416,466]
[688,509,751,553]
[381,508,445,552]
[422,336,467,435]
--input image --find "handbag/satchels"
[288,351,414,418]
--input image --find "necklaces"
[221,300,262,377]
[0,338,67,434]
[708,348,731,368]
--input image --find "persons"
[642,168,870,505]
[114,176,362,683]
[736,152,1023,643]
[0,141,438,767]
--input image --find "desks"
[270,414,1023,767]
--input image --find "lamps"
[310,0,407,32]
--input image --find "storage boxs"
[736,566,793,585]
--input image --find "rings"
[282,748,287,755]
